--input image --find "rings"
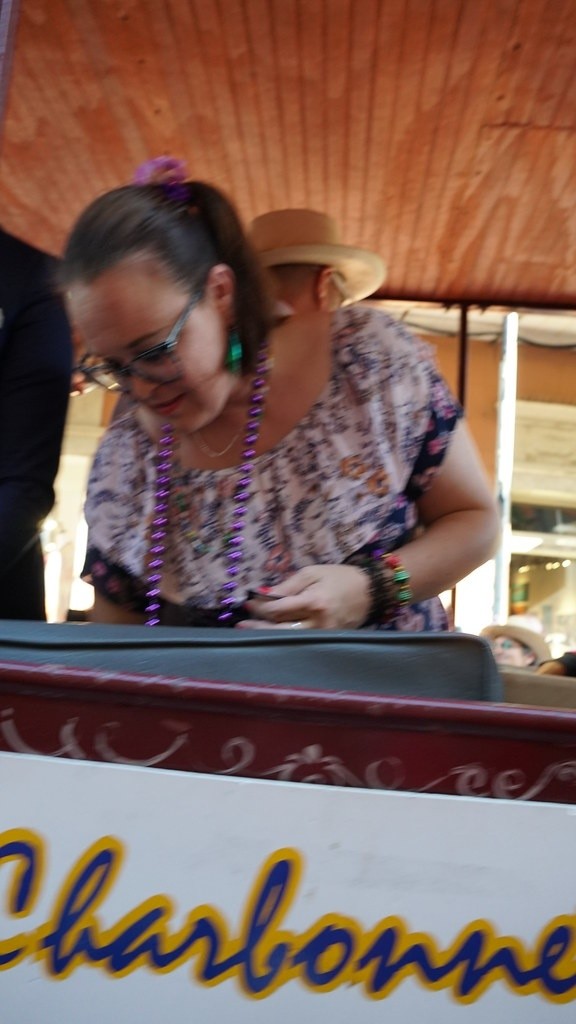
[292,621,301,629]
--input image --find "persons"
[477,624,552,672]
[0,228,74,620]
[247,207,387,316]
[58,156,504,631]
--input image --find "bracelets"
[348,554,390,630]
[378,553,411,613]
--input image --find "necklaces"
[146,345,268,629]
[192,416,246,457]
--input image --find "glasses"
[332,271,352,307]
[78,277,205,391]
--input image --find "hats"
[252,209,386,308]
